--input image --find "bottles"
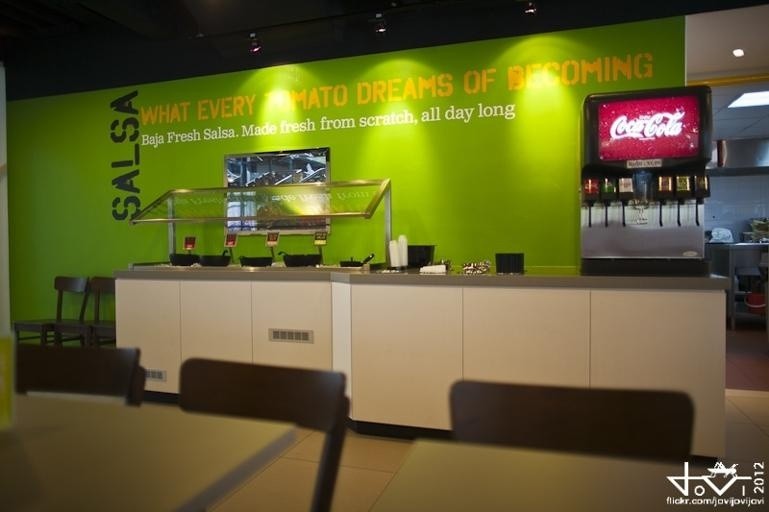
[390,236,409,268]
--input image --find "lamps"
[243,0,539,58]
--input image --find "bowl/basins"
[408,245,435,267]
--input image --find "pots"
[338,254,373,267]
[167,250,320,266]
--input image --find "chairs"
[445,377,694,466]
[14,346,151,406]
[10,276,89,347]
[53,275,116,348]
[177,354,351,511]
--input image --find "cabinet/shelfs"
[710,242,768,331]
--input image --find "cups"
[495,252,524,275]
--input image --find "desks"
[1,382,300,510]
[365,436,768,512]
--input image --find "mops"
[389,234,409,268]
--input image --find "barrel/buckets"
[744,293,765,313]
